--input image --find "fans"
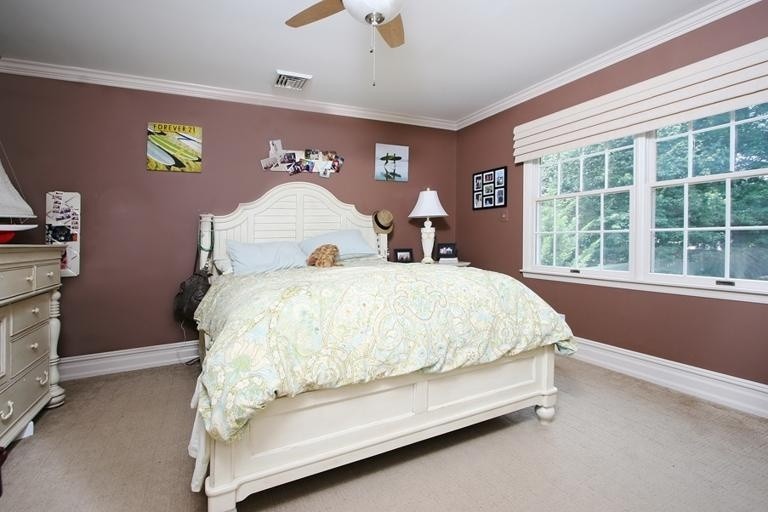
[285,1,408,49]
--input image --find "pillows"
[225,228,378,276]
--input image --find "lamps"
[340,1,414,89]
[408,186,450,265]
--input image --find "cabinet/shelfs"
[0,244,68,444]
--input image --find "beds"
[199,181,559,511]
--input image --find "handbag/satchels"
[174,270,212,329]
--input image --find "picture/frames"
[393,248,414,263]
[437,243,456,259]
[472,166,507,209]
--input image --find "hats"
[372,208,393,234]
